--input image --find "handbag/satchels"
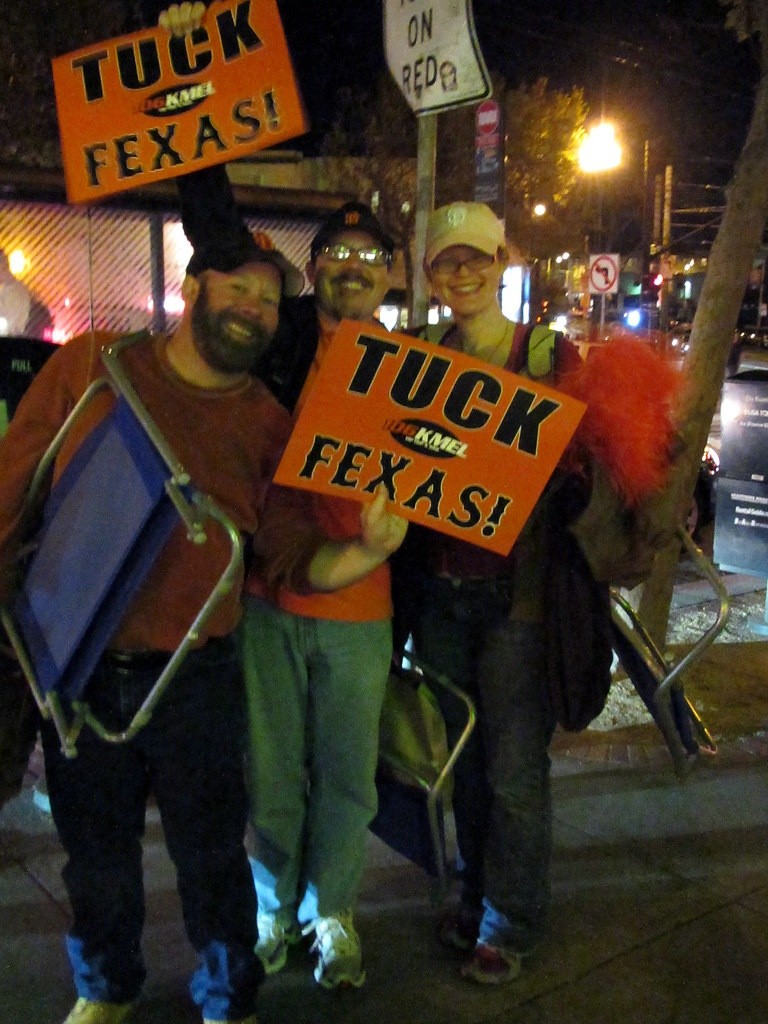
[552,537,614,735]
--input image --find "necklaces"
[461,317,509,363]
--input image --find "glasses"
[314,244,393,266]
[433,252,497,273]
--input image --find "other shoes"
[436,901,484,953]
[62,995,138,1024]
[457,936,541,984]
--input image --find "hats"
[308,200,395,271]
[424,200,506,268]
[185,240,305,301]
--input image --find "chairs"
[0,325,245,759]
[609,523,732,777]
[366,653,477,909]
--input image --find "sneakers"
[306,906,366,990]
[255,910,304,974]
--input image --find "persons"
[152,1,396,994]
[0,201,592,1024]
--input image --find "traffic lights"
[640,272,664,303]
